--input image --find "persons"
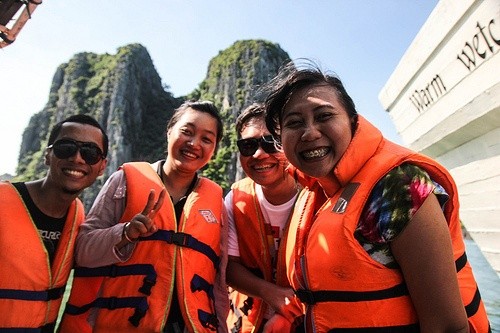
[255,58,492,333]
[56,101,231,333]
[1,113,109,332]
[219,101,308,333]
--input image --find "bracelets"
[123,222,137,242]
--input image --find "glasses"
[46,136,106,165]
[237,134,283,157]
